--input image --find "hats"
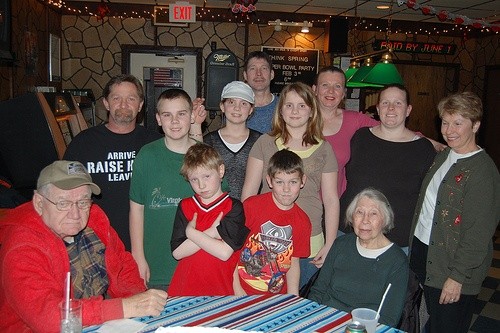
[37,161,101,195]
[221,81,256,104]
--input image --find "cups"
[351,308,380,333]
[58,298,83,333]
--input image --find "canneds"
[344,322,367,333]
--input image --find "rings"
[450,298,455,301]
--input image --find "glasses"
[38,192,93,210]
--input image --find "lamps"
[274,19,283,31]
[300,21,309,33]
[344,59,365,88]
[345,57,385,88]
[361,52,406,86]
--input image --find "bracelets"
[189,133,203,137]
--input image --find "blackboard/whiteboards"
[261,46,320,96]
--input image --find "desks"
[82,294,409,333]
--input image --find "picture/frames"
[49,34,62,83]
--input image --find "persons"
[240,80,340,296]
[242,51,280,135]
[407,90,500,333]
[306,188,411,329]
[312,66,448,201]
[335,83,437,257]
[204,80,263,204]
[128,88,231,292]
[59,73,207,253]
[166,141,245,296]
[0,160,168,333]
[231,149,312,295]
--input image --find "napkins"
[94,317,150,333]
